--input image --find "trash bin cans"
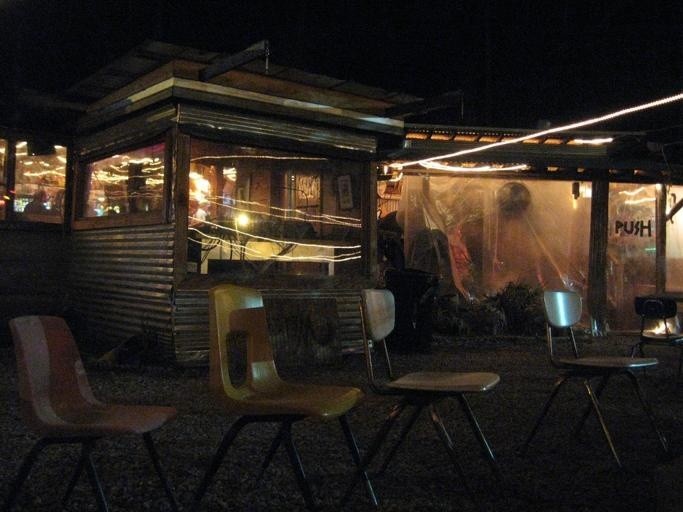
[384,268,440,356]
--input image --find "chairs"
[186,283,381,512]
[332,287,509,512]
[0,315,180,512]
[633,295,683,379]
[518,289,672,484]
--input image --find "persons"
[96,203,109,216]
[23,189,50,214]
[189,199,210,223]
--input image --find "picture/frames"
[336,173,356,211]
